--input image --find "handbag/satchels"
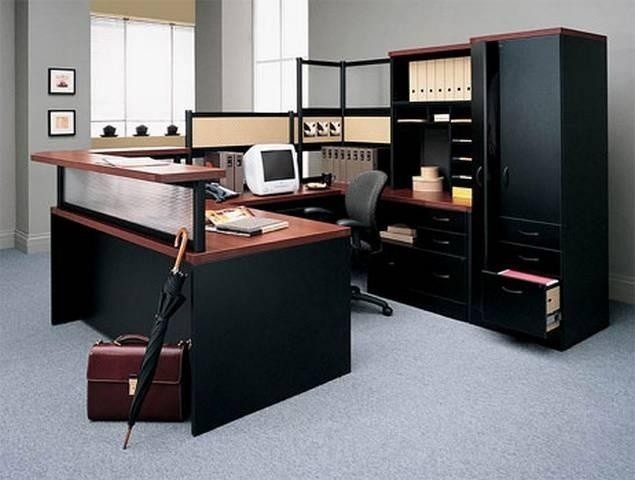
[87,334,193,421]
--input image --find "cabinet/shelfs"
[371,193,474,324]
[390,40,473,191]
[472,27,609,350]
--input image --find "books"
[102,156,174,168]
[379,230,418,244]
[205,204,256,232]
[216,216,289,237]
[386,223,416,238]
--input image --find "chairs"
[301,170,396,320]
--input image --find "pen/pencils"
[247,208,255,217]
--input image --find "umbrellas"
[120,226,190,450]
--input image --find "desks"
[53,177,347,439]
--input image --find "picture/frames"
[47,107,75,136]
[47,65,76,95]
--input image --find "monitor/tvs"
[243,143,299,195]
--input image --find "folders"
[408,56,472,102]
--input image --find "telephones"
[204,183,233,203]
